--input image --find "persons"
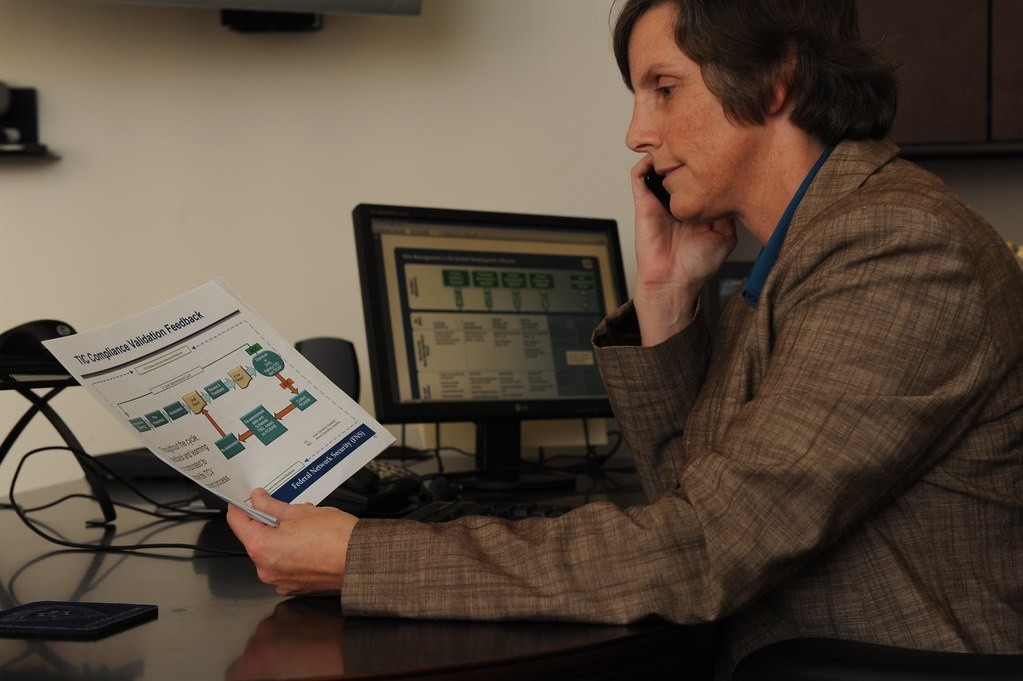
[225,1,1023,655]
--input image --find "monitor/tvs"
[352,203,630,504]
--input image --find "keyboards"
[403,500,573,523]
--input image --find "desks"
[0,443,645,681]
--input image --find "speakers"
[296,337,360,404]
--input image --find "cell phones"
[643,166,673,212]
